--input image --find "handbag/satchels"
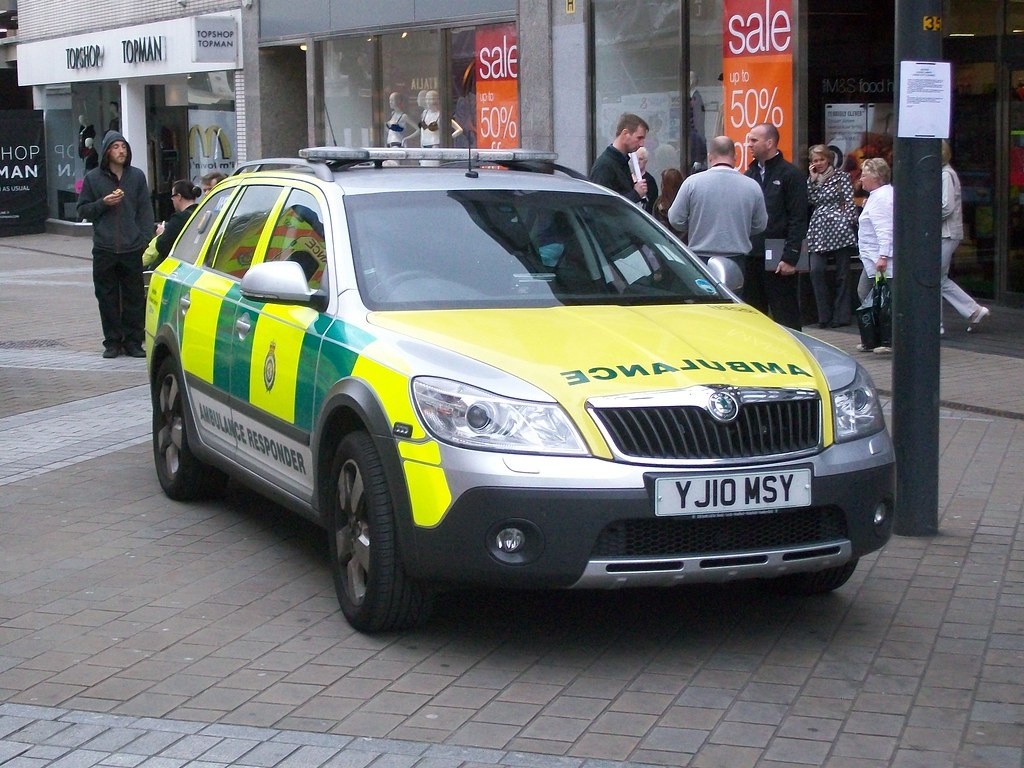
[141,232,163,266]
[855,269,893,347]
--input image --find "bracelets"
[881,256,888,259]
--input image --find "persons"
[588,111,649,203]
[653,168,688,245]
[141,171,228,271]
[689,70,707,172]
[806,145,858,330]
[384,89,462,167]
[668,135,768,297]
[78,115,96,159]
[83,137,99,174]
[108,101,119,132]
[77,131,154,358]
[941,138,990,336]
[856,157,893,354]
[628,147,659,214]
[743,122,808,333]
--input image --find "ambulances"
[141,146,897,637]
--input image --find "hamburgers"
[112,189,124,197]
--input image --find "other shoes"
[831,323,846,328]
[873,347,893,354]
[966,307,990,332]
[125,346,148,358]
[855,344,872,351]
[817,322,827,328]
[103,346,122,358]
